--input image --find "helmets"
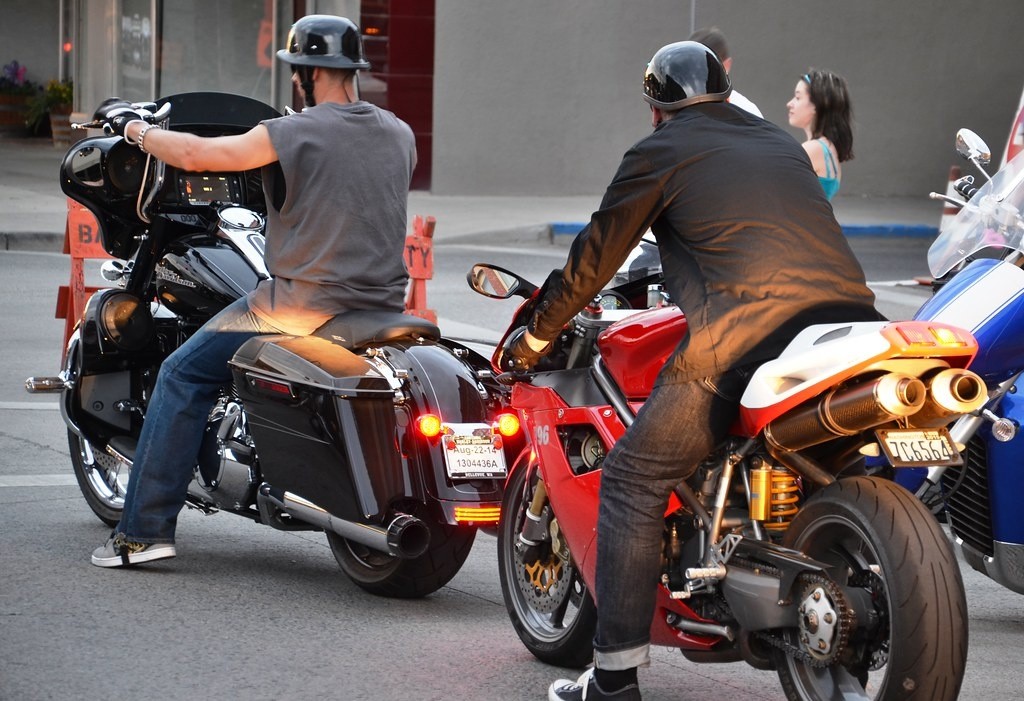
[277,14,371,69]
[643,41,733,109]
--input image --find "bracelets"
[137,125,159,153]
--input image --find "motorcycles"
[464,231,970,701]
[24,89,520,600]
[843,126,1024,597]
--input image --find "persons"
[91,13,417,567]
[503,30,890,701]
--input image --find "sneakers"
[92,532,177,567]
[548,666,642,701]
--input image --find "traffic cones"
[919,164,964,288]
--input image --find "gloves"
[93,97,146,145]
[500,333,552,374]
